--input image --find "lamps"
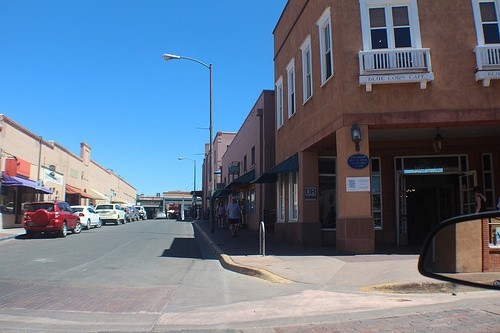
[351,122,362,152]
[432,127,444,149]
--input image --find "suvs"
[22,200,82,238]
[94,203,127,225]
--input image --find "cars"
[69,205,102,230]
[0,202,14,214]
[120,205,147,223]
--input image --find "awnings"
[249,152,298,184]
[2,173,53,194]
[216,170,254,197]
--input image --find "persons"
[473,186,487,214]
[239,204,245,225]
[214,201,225,229]
[225,197,240,237]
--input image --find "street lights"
[176,156,196,221]
[163,53,216,234]
